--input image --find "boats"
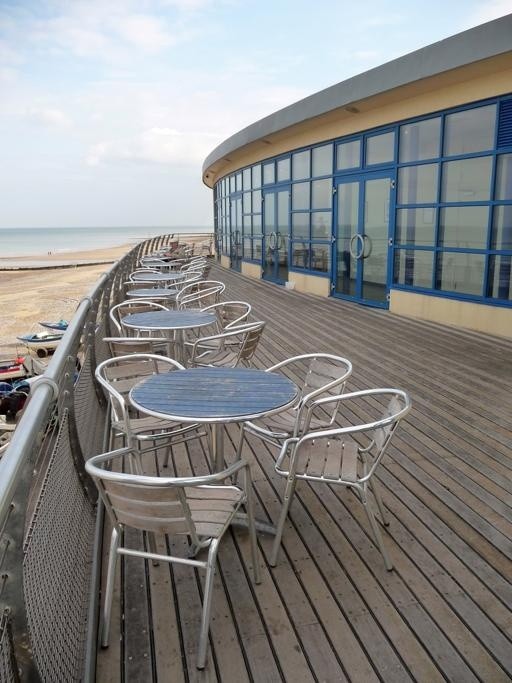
[0,319,71,421]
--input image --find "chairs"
[93,353,217,474]
[235,351,353,485]
[269,388,414,572]
[102,238,265,373]
[253,243,388,282]
[82,441,262,670]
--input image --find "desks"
[126,370,298,546]
